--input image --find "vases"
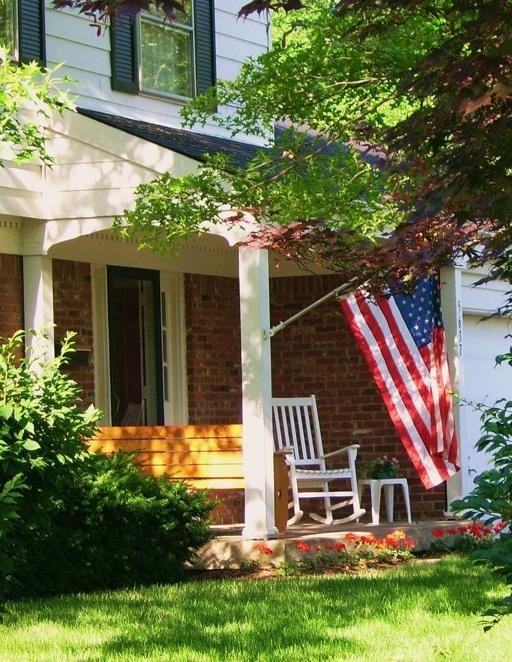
[376,473,389,479]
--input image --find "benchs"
[80,423,245,490]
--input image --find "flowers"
[363,455,401,479]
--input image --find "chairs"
[272,394,367,526]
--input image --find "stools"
[356,478,412,526]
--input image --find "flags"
[339,264,461,490]
